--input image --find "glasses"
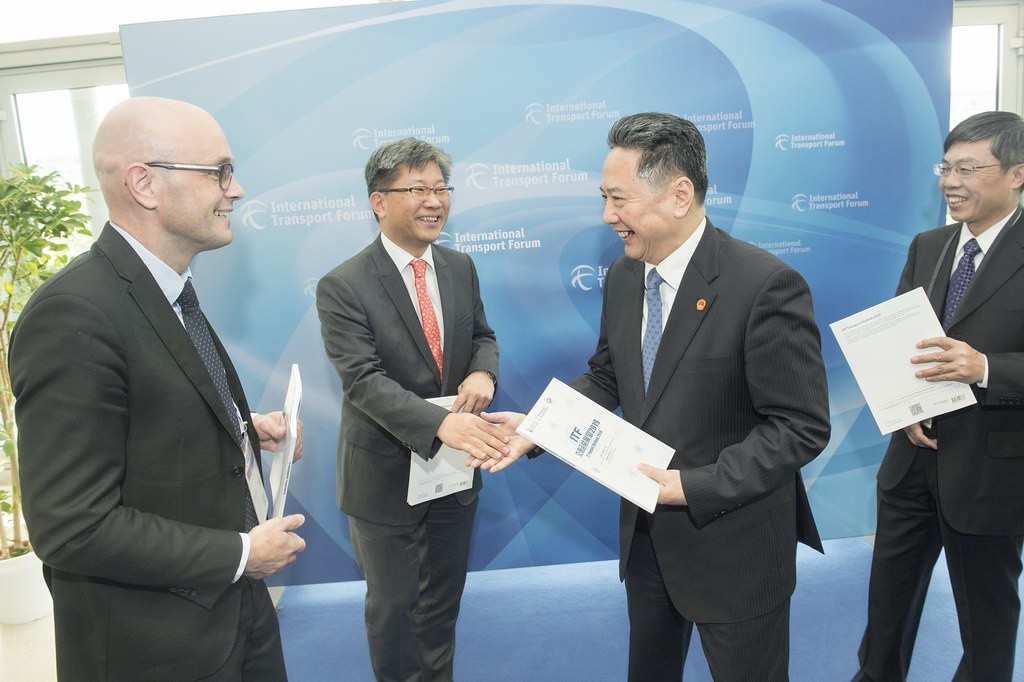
[933,162,1021,178]
[377,185,454,201]
[125,162,234,190]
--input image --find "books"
[515,375,677,514]
[829,285,978,435]
[269,363,303,518]
[407,395,475,507]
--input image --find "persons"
[314,137,510,682]
[843,110,1024,681]
[6,94,306,682]
[467,111,832,682]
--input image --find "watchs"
[484,370,496,384]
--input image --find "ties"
[642,267,665,397]
[178,277,258,534]
[411,259,444,384]
[942,239,982,330]
